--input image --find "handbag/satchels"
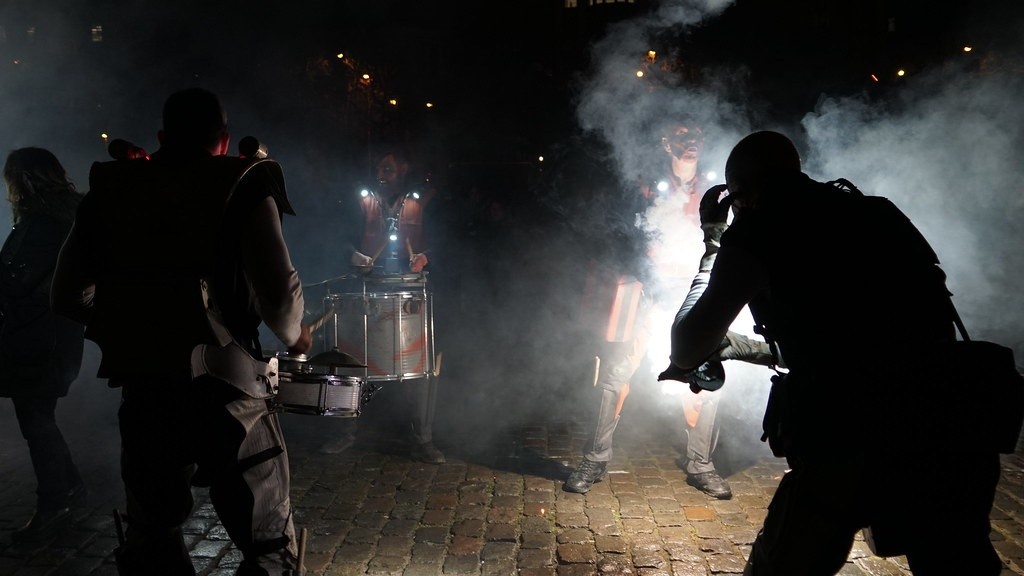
[578,256,643,346]
[929,337,1024,455]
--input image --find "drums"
[316,272,435,381]
[258,349,385,420]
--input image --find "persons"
[47,86,313,575]
[670,131,1002,576]
[318,149,448,465]
[0,147,84,543]
[561,113,733,500]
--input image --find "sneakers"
[565,456,608,493]
[316,428,354,454]
[9,492,84,542]
[410,441,446,465]
[685,470,732,497]
